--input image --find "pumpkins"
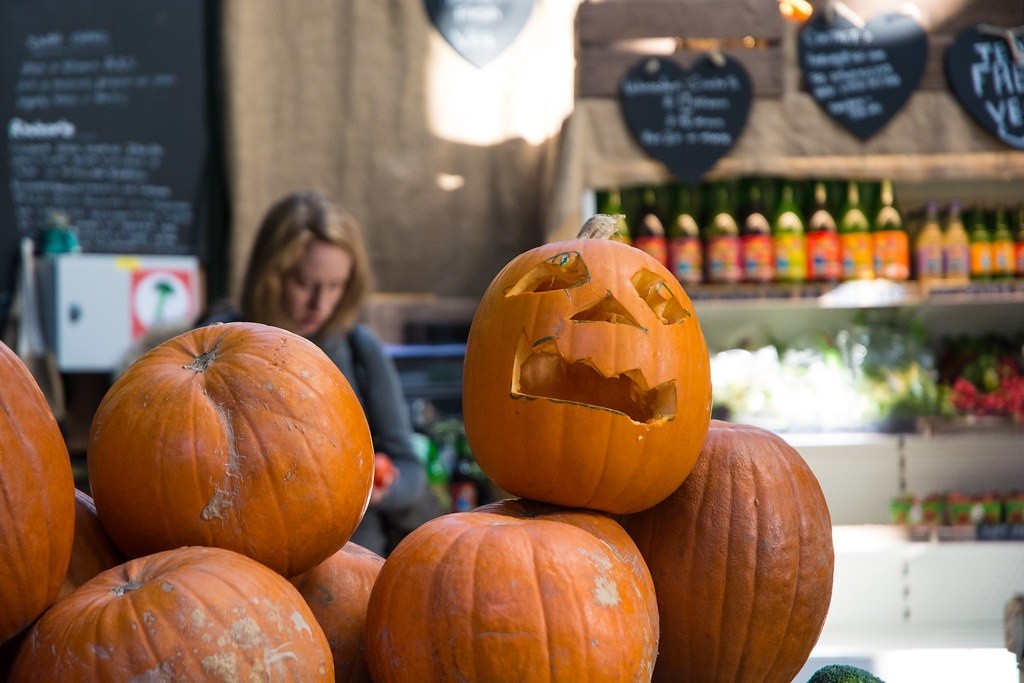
[0,321,834,683]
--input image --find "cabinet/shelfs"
[554,151,1023,435]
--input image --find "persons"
[201,192,425,557]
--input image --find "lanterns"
[461,237,714,514]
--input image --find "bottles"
[704,187,744,282]
[838,180,874,281]
[914,199,1024,294]
[805,182,841,280]
[873,179,910,280]
[635,186,671,271]
[774,182,806,286]
[741,185,776,283]
[602,186,633,246]
[673,184,705,285]
